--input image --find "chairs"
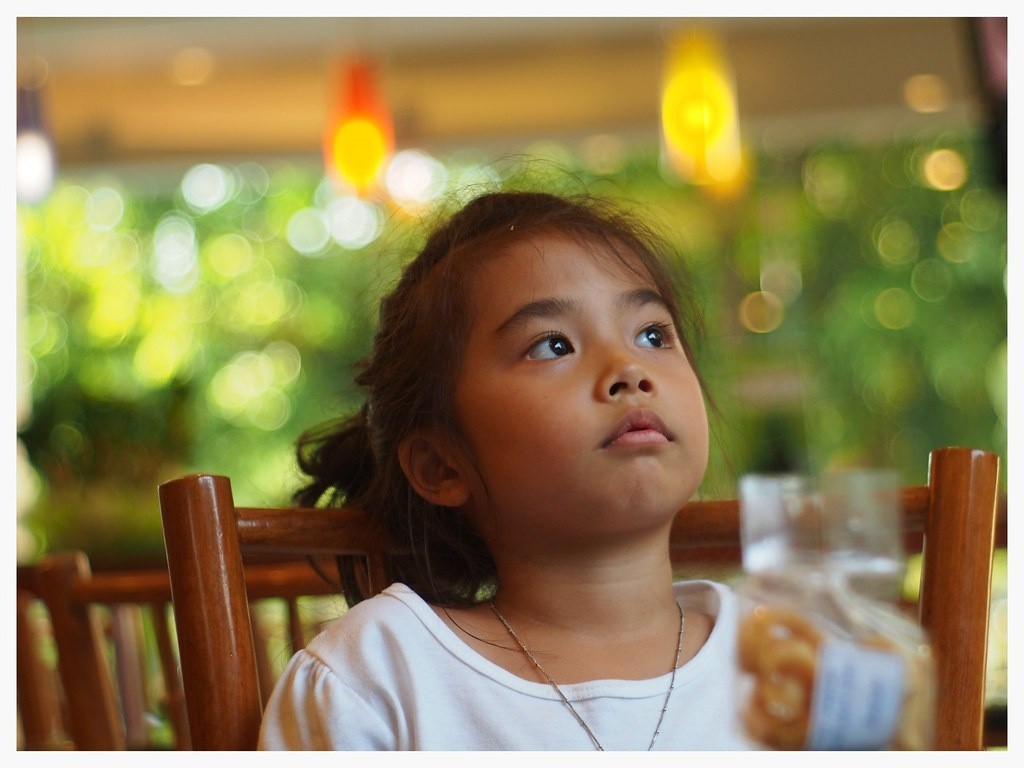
[17,445,999,751]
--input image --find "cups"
[740,470,906,611]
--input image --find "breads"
[734,604,937,751]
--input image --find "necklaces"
[489,595,684,752]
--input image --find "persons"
[253,190,824,752]
